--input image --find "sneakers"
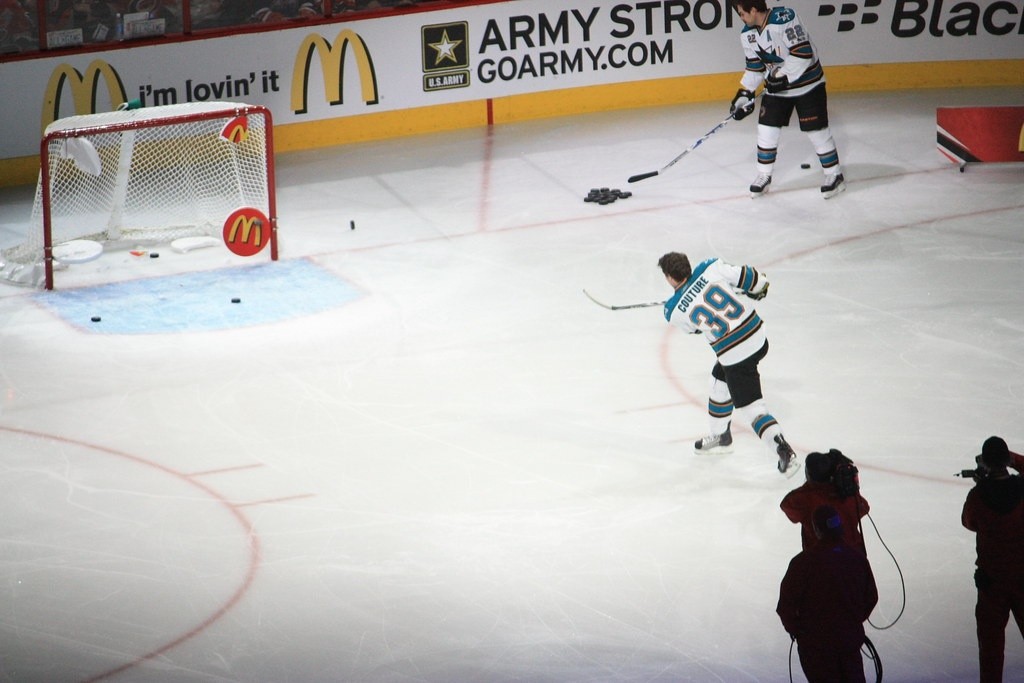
[749,175,774,199]
[774,433,801,481]
[695,421,734,454]
[821,173,847,199]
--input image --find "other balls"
[91,317,101,322]
[583,187,633,205]
[231,298,240,303]
[351,221,355,229]
[801,164,811,169]
[150,253,159,258]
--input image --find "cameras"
[962,454,987,479]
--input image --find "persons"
[16,0,417,55]
[655,251,802,479]
[961,436,1024,683]
[782,449,870,560]
[728,0,849,200]
[776,505,880,683]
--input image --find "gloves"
[764,72,788,93]
[730,88,756,120]
[744,283,771,300]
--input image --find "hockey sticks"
[582,288,669,310]
[628,88,769,184]
